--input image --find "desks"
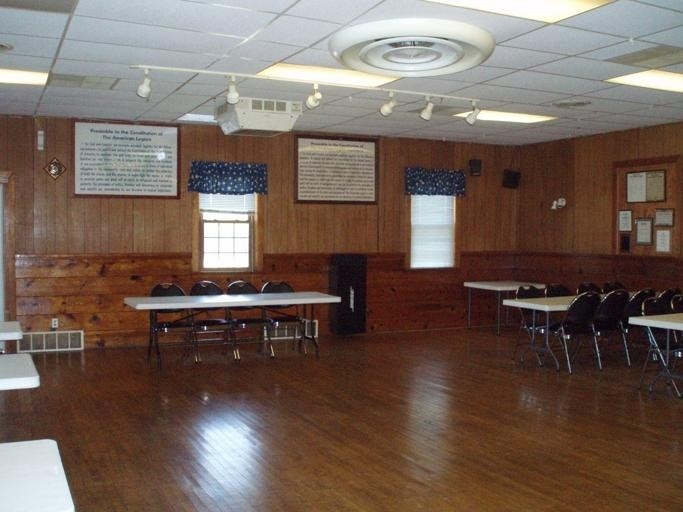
[0,353,40,390]
[459,281,546,337]
[503,287,664,369]
[124,291,342,367]
[0,322,23,341]
[0,438,75,512]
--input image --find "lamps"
[328,18,495,79]
[379,91,397,117]
[129,71,162,100]
[420,95,435,120]
[465,101,480,126]
[303,83,324,111]
[222,76,246,106]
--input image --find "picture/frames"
[654,207,674,227]
[635,217,653,245]
[617,210,633,233]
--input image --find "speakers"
[328,253,366,335]
[470,158,482,176]
[503,169,522,188]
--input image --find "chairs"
[147,279,307,363]
[514,281,627,372]
[544,287,682,374]
